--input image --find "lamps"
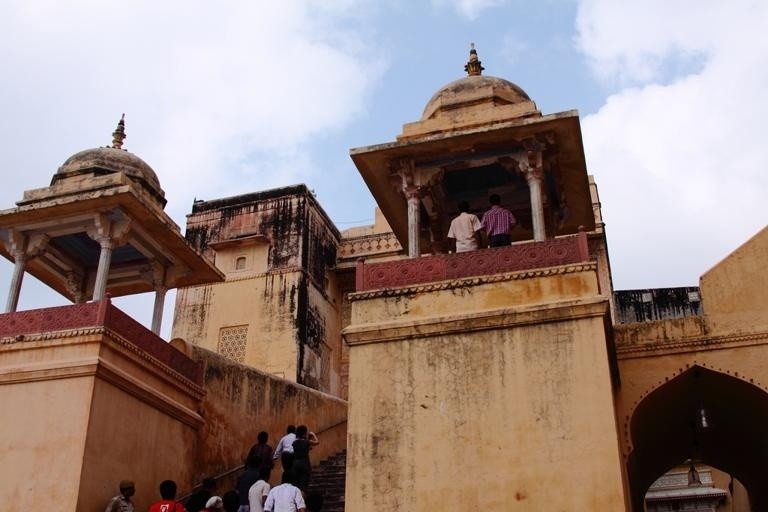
[688,461,702,487]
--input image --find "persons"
[147,479,186,511]
[199,495,225,511]
[222,490,241,511]
[446,199,488,255]
[247,467,272,511]
[481,194,517,249]
[104,478,137,512]
[244,429,276,483]
[273,425,298,484]
[264,469,307,511]
[236,458,262,511]
[291,424,320,498]
[183,475,218,511]
